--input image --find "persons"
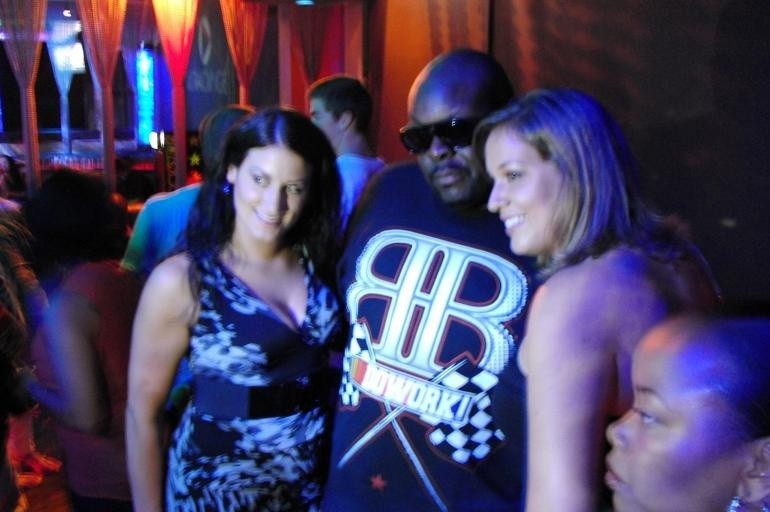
[124,105,352,512]
[0,154,144,510]
[473,82,728,511]
[314,44,551,511]
[309,77,387,243]
[119,105,259,455]
[602,297,770,512]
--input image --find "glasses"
[400,117,475,155]
